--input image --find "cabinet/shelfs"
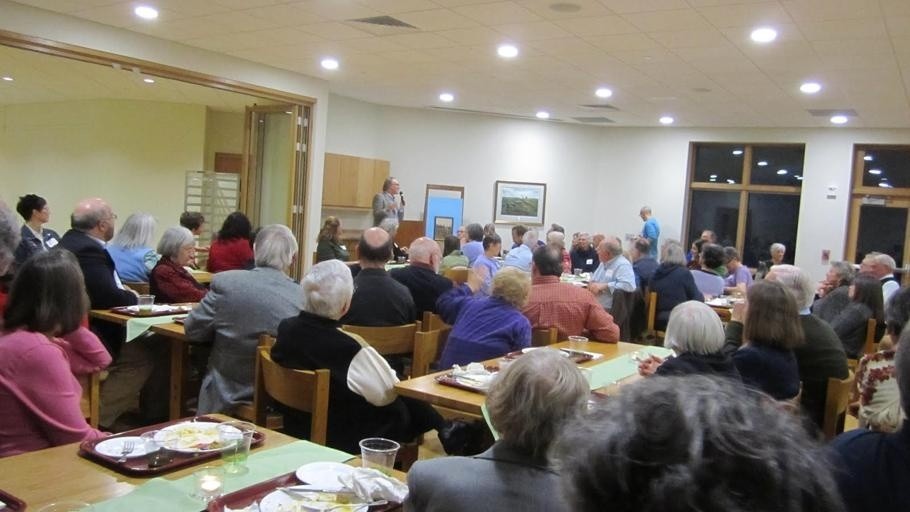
[320,152,391,212]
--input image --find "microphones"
[400,191,406,206]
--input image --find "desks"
[393,220,425,248]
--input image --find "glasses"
[102,215,118,223]
[391,182,400,187]
[456,230,463,233]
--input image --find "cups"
[568,335,588,360]
[195,466,225,498]
[217,421,254,476]
[574,268,582,276]
[138,294,155,315]
[359,439,399,478]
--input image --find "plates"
[128,303,170,313]
[507,351,593,364]
[79,414,265,477]
[434,369,481,393]
[173,318,185,324]
[96,436,160,457]
[295,461,357,486]
[208,470,306,512]
[154,421,241,453]
[259,485,369,512]
[111,304,193,317]
[592,391,607,400]
[522,347,569,359]
[458,370,496,388]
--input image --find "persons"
[434,262,534,372]
[572,233,578,247]
[269,257,478,456]
[632,238,660,291]
[587,239,637,314]
[149,225,212,304]
[689,243,725,300]
[700,230,716,243]
[340,227,417,376]
[206,210,256,273]
[722,246,754,295]
[0,247,113,458]
[548,373,848,511]
[483,223,502,258]
[607,234,633,265]
[471,234,503,298]
[57,196,156,434]
[179,211,211,271]
[547,231,573,273]
[440,235,469,270]
[647,243,713,333]
[372,176,406,229]
[570,232,600,274]
[848,286,906,419]
[808,260,854,313]
[0,200,20,281]
[386,235,454,322]
[591,232,605,248]
[723,263,849,430]
[378,217,410,263]
[104,210,159,283]
[831,271,885,360]
[547,224,564,242]
[860,251,882,276]
[638,207,661,261]
[314,215,351,265]
[457,226,465,239]
[518,244,620,344]
[510,226,546,249]
[756,242,786,281]
[512,225,529,249]
[659,238,682,261]
[870,254,901,311]
[15,193,62,275]
[637,299,744,383]
[733,279,806,401]
[459,223,485,265]
[503,230,540,273]
[406,348,592,512]
[688,238,709,270]
[184,222,308,418]
[825,318,910,512]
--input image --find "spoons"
[302,498,390,510]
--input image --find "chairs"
[74,369,100,429]
[531,327,559,348]
[422,311,447,331]
[127,281,150,295]
[641,290,666,347]
[644,285,650,313]
[235,333,284,431]
[826,357,865,441]
[444,268,469,284]
[412,327,453,379]
[253,344,330,446]
[342,321,422,381]
[865,318,877,356]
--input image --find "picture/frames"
[493,180,547,226]
[433,215,454,241]
